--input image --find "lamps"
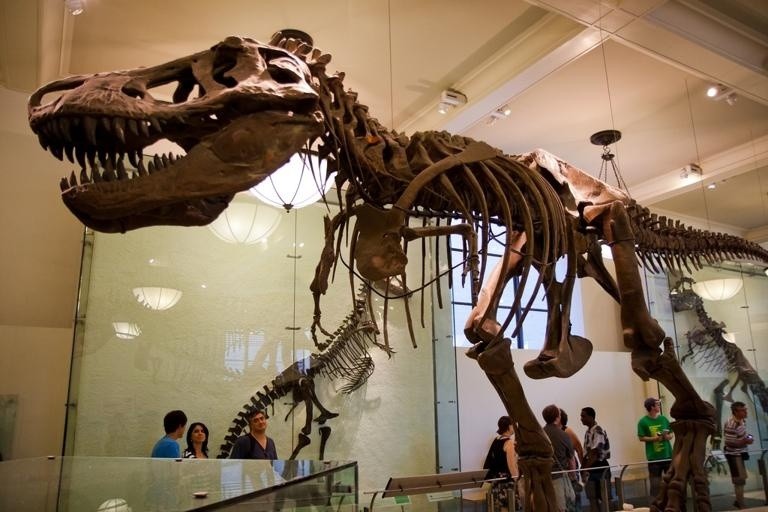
[692,277,744,301]
[114,322,140,340]
[132,285,183,311]
[249,147,337,214]
[207,197,283,245]
[590,128,635,260]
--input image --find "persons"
[480,405,613,512]
[637,398,673,498]
[151,410,187,458]
[230,411,278,459]
[182,423,209,459]
[723,402,754,511]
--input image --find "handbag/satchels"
[564,474,576,500]
